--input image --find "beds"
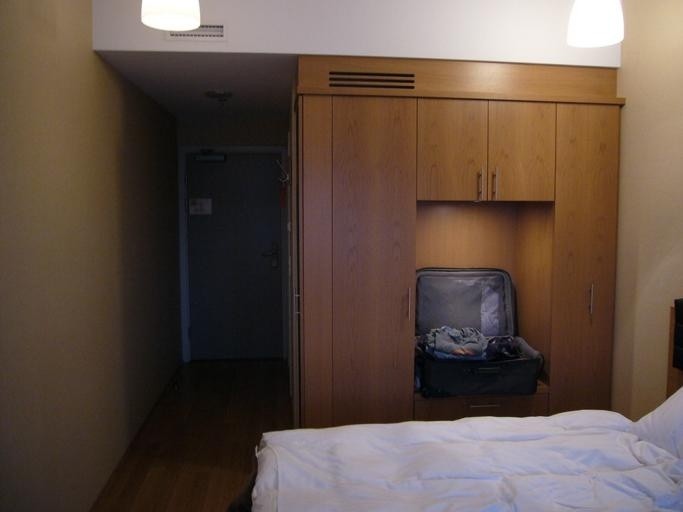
[251,385,683,512]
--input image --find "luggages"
[416,262,541,398]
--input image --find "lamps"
[141,0,200,31]
[567,0,624,48]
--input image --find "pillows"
[622,384,683,460]
